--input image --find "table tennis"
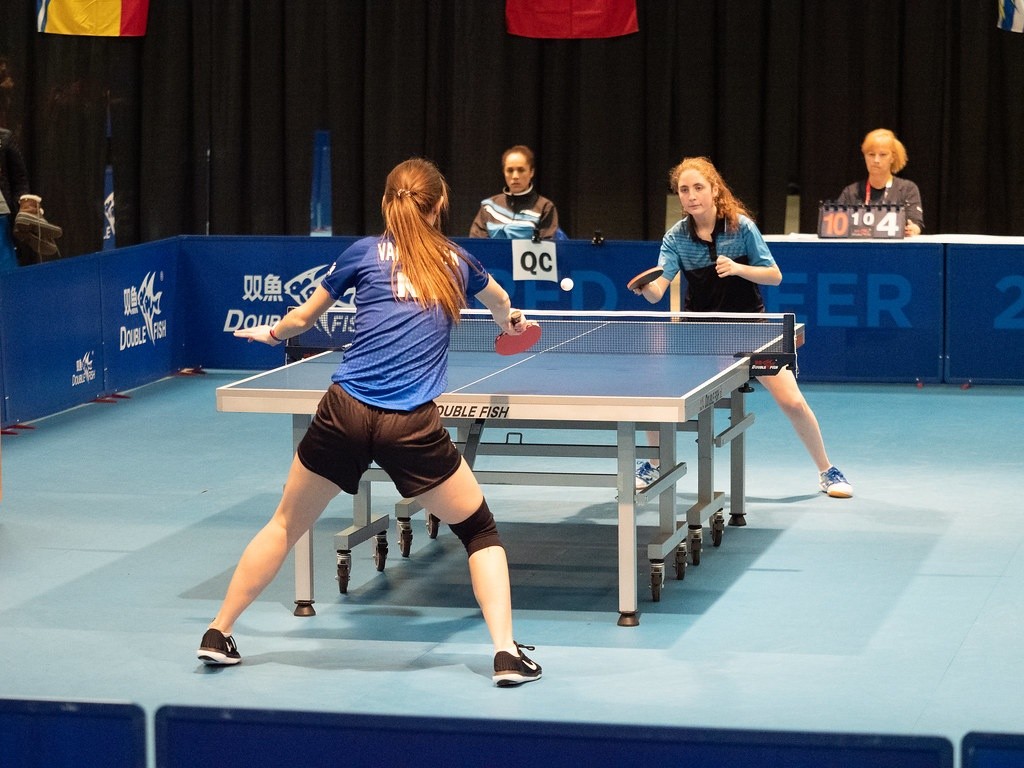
[560,277,574,291]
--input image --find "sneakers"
[197,629,242,664]
[492,641,542,686]
[818,465,853,498]
[636,461,660,489]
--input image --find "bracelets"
[270,321,286,341]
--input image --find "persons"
[468,145,558,239]
[633,158,856,500]
[197,158,544,687]
[835,129,921,239]
[0,129,62,257]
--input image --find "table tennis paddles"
[627,267,664,294]
[495,310,542,356]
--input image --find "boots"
[14,194,62,255]
